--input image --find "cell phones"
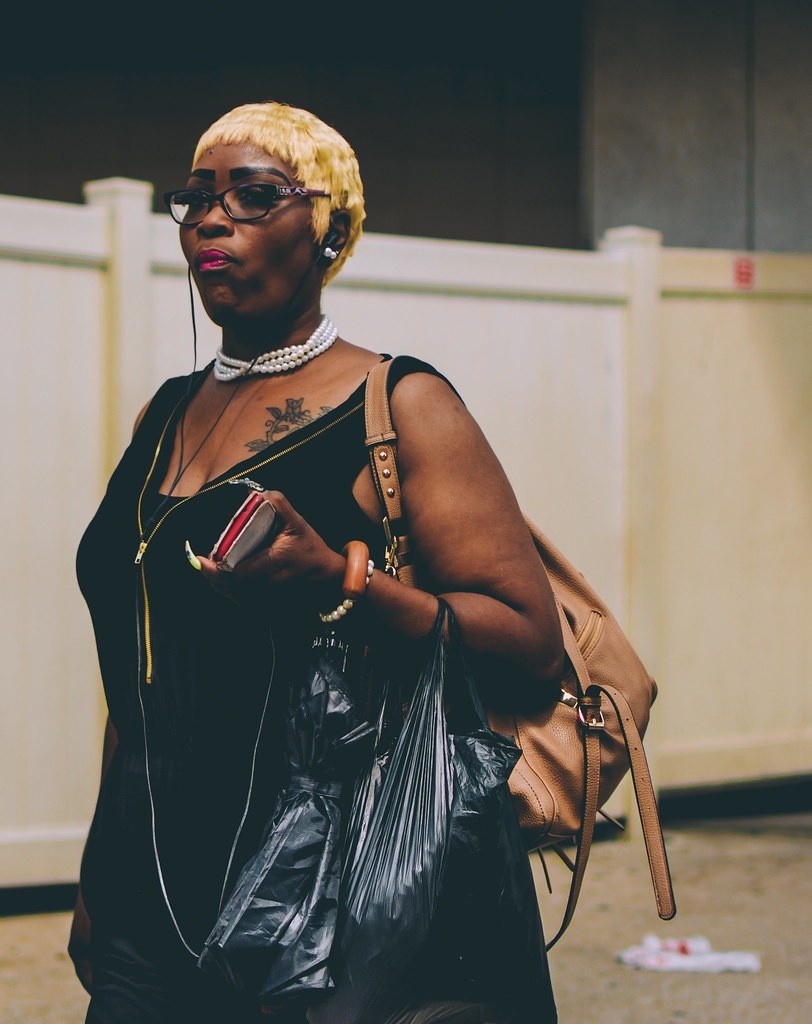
[214,493,264,563]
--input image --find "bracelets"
[320,557,375,624]
[344,539,370,608]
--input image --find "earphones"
[317,232,341,257]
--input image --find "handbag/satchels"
[351,355,659,854]
[327,591,520,977]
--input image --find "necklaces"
[207,311,340,383]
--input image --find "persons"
[70,103,567,1023]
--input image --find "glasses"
[164,183,349,226]
